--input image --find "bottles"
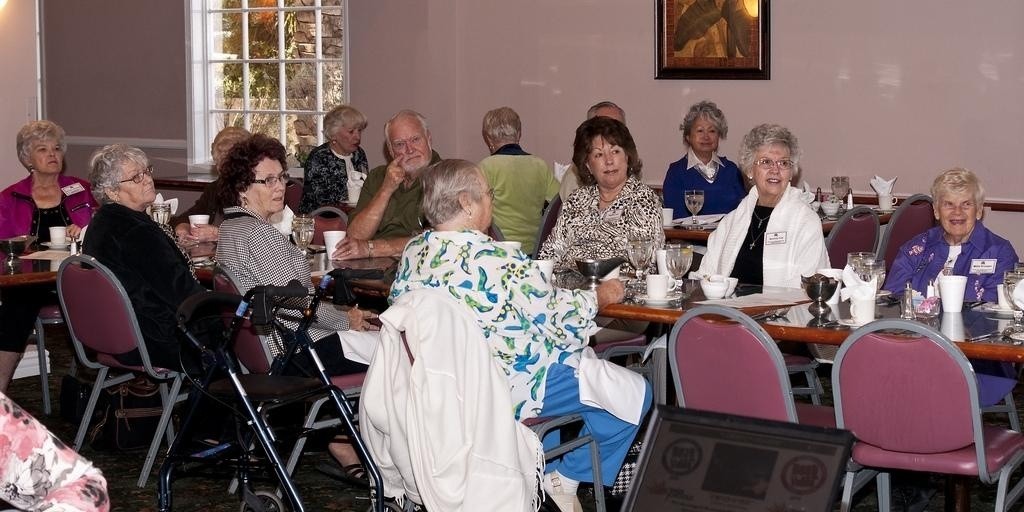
[70,237,77,258]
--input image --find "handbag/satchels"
[86,374,180,452]
[58,374,92,424]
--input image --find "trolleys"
[155,267,388,512]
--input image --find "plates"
[40,240,70,250]
[637,294,678,305]
[342,200,358,208]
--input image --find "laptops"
[619,402,854,511]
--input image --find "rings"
[196,236,198,240]
[346,249,351,254]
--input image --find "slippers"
[314,459,369,485]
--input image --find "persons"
[882,166,1019,410]
[213,134,381,481]
[559,102,627,204]
[536,117,666,277]
[687,124,832,289]
[331,109,442,261]
[474,106,561,258]
[662,101,747,219]
[171,127,251,241]
[387,159,652,511]
[298,105,370,220]
[0,120,100,394]
[79,146,254,461]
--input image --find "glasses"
[488,188,495,201]
[119,165,154,183]
[250,173,290,187]
[753,158,794,170]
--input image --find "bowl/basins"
[799,275,840,304]
[0,257,28,277]
[0,235,38,259]
[573,257,622,283]
[801,302,836,330]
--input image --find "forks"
[750,303,794,322]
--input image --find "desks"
[0,224,1024,363]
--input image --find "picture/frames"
[651,0,772,82]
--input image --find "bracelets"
[367,240,373,258]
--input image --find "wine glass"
[684,189,705,229]
[664,242,694,298]
[626,237,654,288]
[290,214,315,260]
[150,202,172,226]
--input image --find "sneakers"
[541,471,583,512]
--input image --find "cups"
[655,250,671,275]
[645,274,677,299]
[661,209,674,226]
[598,264,621,282]
[49,226,67,244]
[497,240,522,252]
[845,252,1024,346]
[49,259,66,272]
[322,231,348,261]
[346,187,360,201]
[188,214,211,227]
[816,268,844,306]
[698,276,738,301]
[530,260,555,284]
[809,175,899,219]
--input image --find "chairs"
[668,305,838,431]
[204,263,367,510]
[486,185,563,260]
[875,194,937,278]
[275,177,350,246]
[23,252,198,494]
[384,288,652,510]
[829,319,1020,510]
[822,203,881,274]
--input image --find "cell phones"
[307,245,327,253]
